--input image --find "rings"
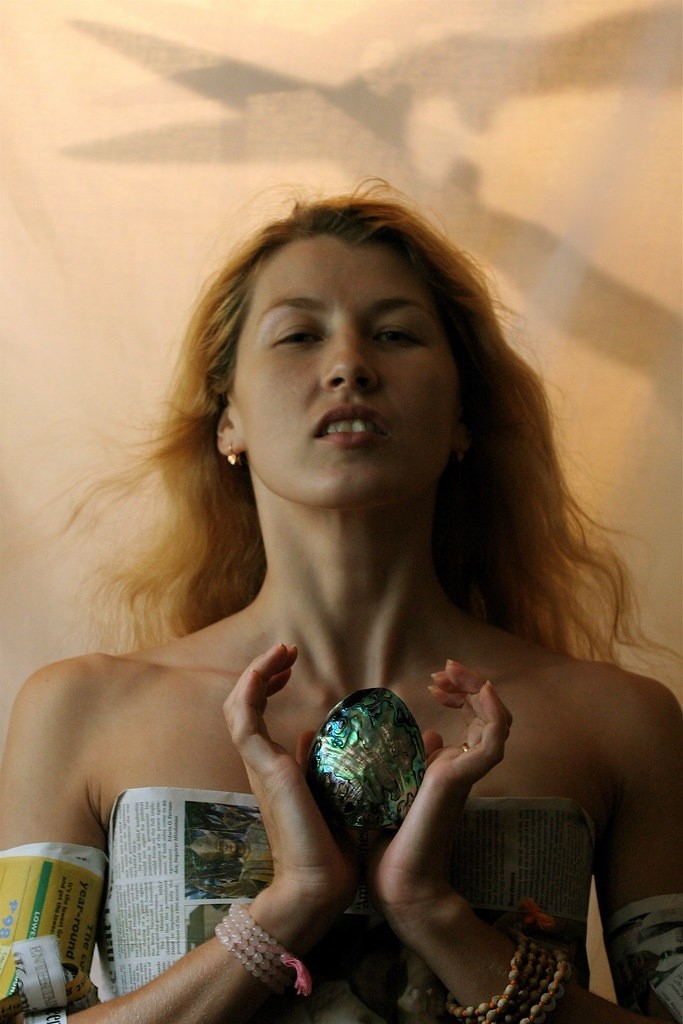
[462,743,470,752]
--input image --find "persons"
[192,816,275,887]
[0,197,683,1024]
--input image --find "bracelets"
[445,897,572,1024]
[213,900,313,998]
